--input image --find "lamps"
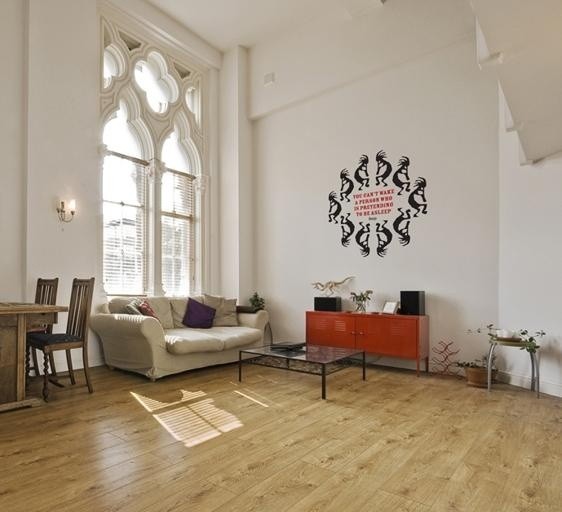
[57,197,78,222]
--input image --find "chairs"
[26,278,97,402]
[26,276,60,388]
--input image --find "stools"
[487,336,540,398]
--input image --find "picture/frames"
[382,300,399,315]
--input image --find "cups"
[495,328,519,337]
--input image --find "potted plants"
[350,290,373,313]
[458,357,496,389]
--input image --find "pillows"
[140,300,156,317]
[202,294,238,326]
[127,298,147,314]
[182,297,218,330]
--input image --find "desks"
[0,302,68,415]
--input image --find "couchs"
[91,295,274,381]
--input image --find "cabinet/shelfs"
[306,309,429,377]
